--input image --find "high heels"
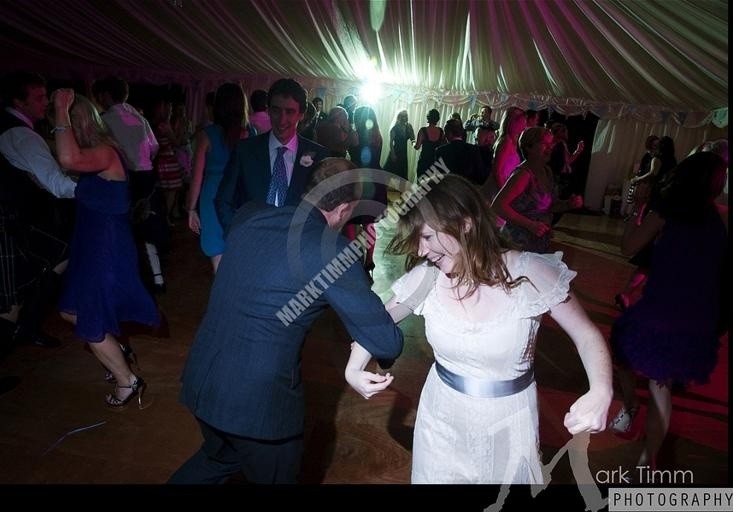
[607,395,641,436]
[619,462,659,487]
[612,292,631,312]
[101,373,149,409]
[101,340,139,382]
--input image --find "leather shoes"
[150,273,169,297]
[14,327,62,348]
[0,370,23,398]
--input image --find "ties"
[262,146,290,210]
